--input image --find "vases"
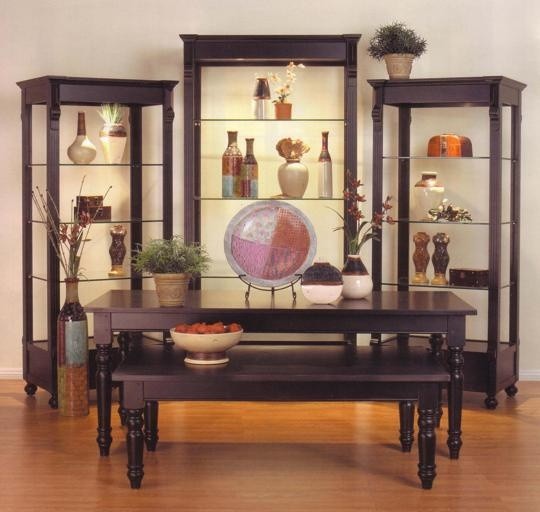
[318,130,332,197]
[108,225,125,276]
[338,256,374,300]
[431,233,451,284]
[67,112,97,163]
[242,138,259,198]
[57,278,90,419]
[413,231,432,284]
[275,104,292,120]
[413,172,445,221]
[277,159,310,198]
[221,131,242,198]
[301,263,344,304]
[253,77,271,101]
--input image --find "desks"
[81,289,477,459]
[110,343,451,490]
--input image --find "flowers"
[268,61,305,103]
[30,175,115,279]
[325,171,397,254]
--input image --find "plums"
[175,321,242,334]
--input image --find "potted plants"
[366,18,428,80]
[126,234,213,307]
[96,102,127,164]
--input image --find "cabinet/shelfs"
[367,74,528,410]
[15,75,180,409]
[178,31,362,291]
